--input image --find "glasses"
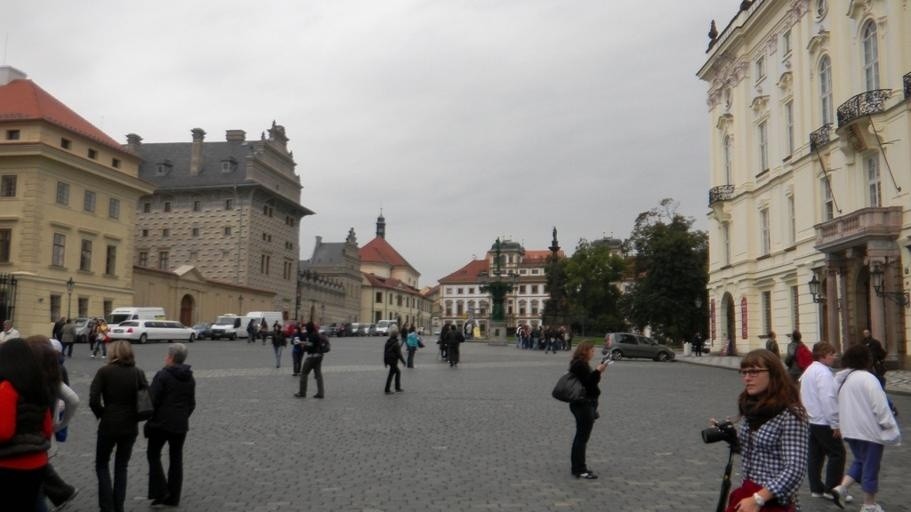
[739,369,768,376]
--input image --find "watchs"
[753,492,765,506]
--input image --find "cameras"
[702,419,736,444]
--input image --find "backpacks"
[319,336,330,353]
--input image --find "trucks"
[101,306,166,329]
[247,310,284,337]
[208,314,256,340]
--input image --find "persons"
[516,324,573,355]
[830,344,902,511]
[798,342,853,502]
[89,340,150,512]
[144,342,195,506]
[247,317,330,398]
[765,331,781,358]
[861,329,883,372]
[569,341,608,479]
[0,316,111,512]
[694,331,702,357]
[787,330,803,381]
[711,349,810,511]
[383,323,464,394]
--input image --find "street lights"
[550,224,564,331]
[695,295,705,357]
[67,275,76,321]
[478,238,521,323]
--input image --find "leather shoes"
[575,469,598,479]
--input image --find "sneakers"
[91,354,107,359]
[292,373,297,376]
[811,485,884,512]
[151,492,167,505]
[50,489,80,512]
[396,390,404,392]
[295,394,306,397]
[386,392,394,395]
[314,395,324,398]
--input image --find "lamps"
[869,265,910,308]
[808,275,827,306]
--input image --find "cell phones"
[605,360,610,364]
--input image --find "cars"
[435,329,441,336]
[66,319,308,342]
[704,337,711,354]
[320,318,398,337]
[602,333,676,361]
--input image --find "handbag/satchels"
[137,389,154,422]
[551,372,589,403]
[1,397,50,460]
